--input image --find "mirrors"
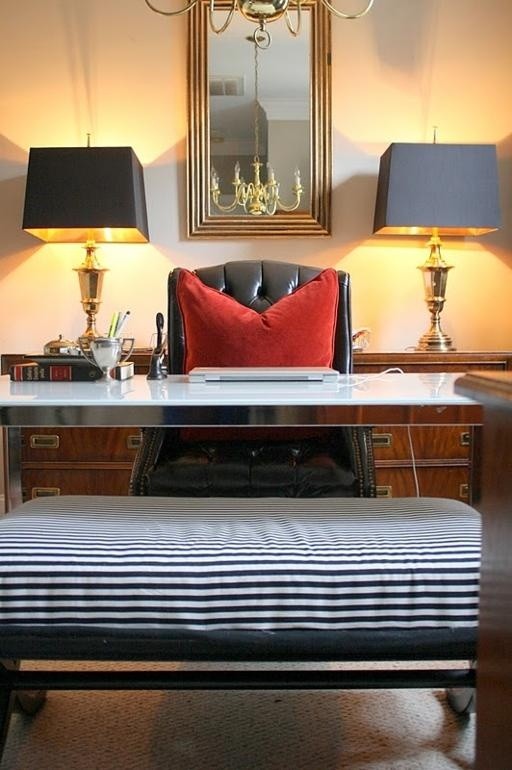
[186,1,333,240]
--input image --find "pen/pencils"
[108,311,131,337]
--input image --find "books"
[11,363,134,381]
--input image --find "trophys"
[78,338,135,384]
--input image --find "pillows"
[171,264,343,379]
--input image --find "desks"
[1,368,487,516]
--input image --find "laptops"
[188,366,340,383]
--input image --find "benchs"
[2,492,480,739]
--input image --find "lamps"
[18,129,151,357]
[371,125,504,352]
[210,35,304,217]
[146,1,374,49]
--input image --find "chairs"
[129,255,378,498]
